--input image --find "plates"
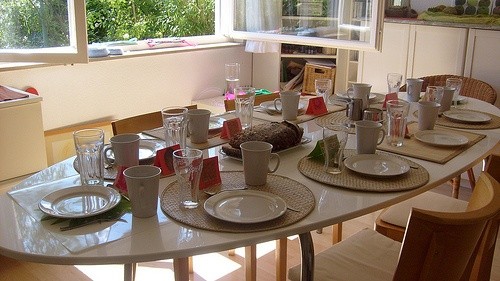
[218,136,312,162]
[39,86,492,224]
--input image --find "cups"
[240,141,281,187]
[123,165,161,219]
[274,91,301,120]
[418,101,441,131]
[323,125,349,175]
[446,78,464,101]
[234,87,255,130]
[345,82,383,120]
[413,101,441,130]
[224,63,240,104]
[173,148,204,210]
[406,79,424,102]
[386,100,410,146]
[185,109,212,143]
[354,121,386,153]
[387,73,403,94]
[105,133,140,166]
[315,78,332,109]
[73,128,105,185]
[161,105,188,155]
[425,86,444,104]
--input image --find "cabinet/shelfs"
[0,85,48,193]
[252,0,373,95]
[357,21,468,93]
[463,28,499,109]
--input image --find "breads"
[221,119,304,157]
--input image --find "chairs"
[111,93,280,281]
[399,75,496,199]
[0,253,93,281]
[372,154,500,243]
[288,171,500,281]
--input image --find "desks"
[0,92,499,281]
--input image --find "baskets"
[301,64,336,97]
